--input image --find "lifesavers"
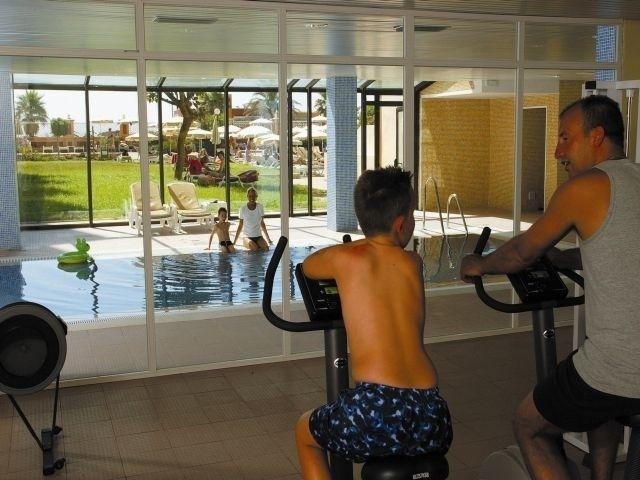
[57,238,94,265]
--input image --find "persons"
[294,167,453,479]
[233,187,274,253]
[204,207,235,253]
[343,234,351,243]
[184,145,224,179]
[461,94,640,479]
[121,150,128,156]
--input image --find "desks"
[197,199,228,224]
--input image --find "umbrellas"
[124,115,329,160]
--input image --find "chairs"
[127,151,259,190]
[122,180,215,235]
